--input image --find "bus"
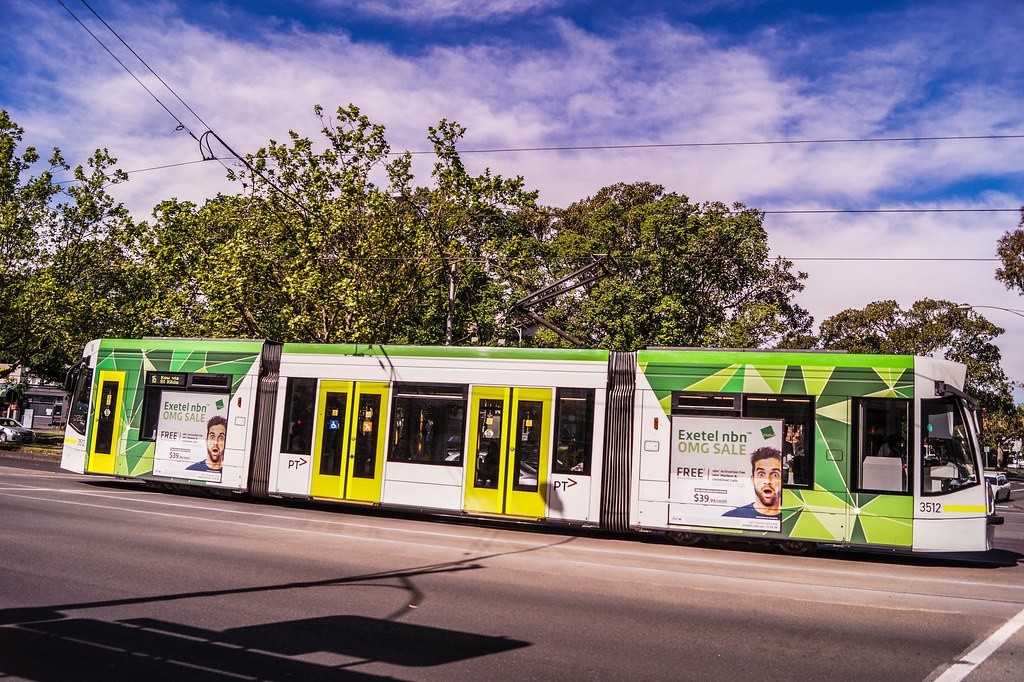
[61,252,1004,563]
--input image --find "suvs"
[984,472,1011,502]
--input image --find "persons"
[185,415,228,473]
[924,436,959,492]
[721,446,781,520]
[877,434,907,490]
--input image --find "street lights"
[390,192,457,346]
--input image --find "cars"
[0,417,36,442]
